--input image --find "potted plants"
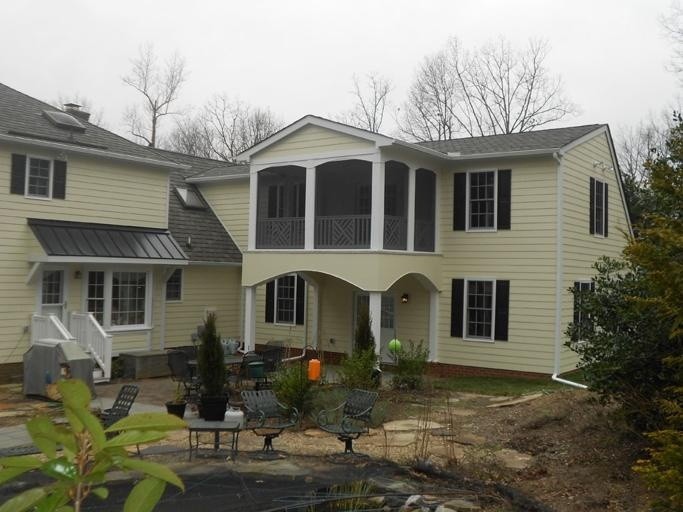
[191,310,229,414]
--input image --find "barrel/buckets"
[307,358,321,381]
[183,403,200,418]
[225,406,244,432]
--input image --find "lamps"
[399,292,408,304]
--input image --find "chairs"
[166,332,380,465]
[87,384,142,456]
